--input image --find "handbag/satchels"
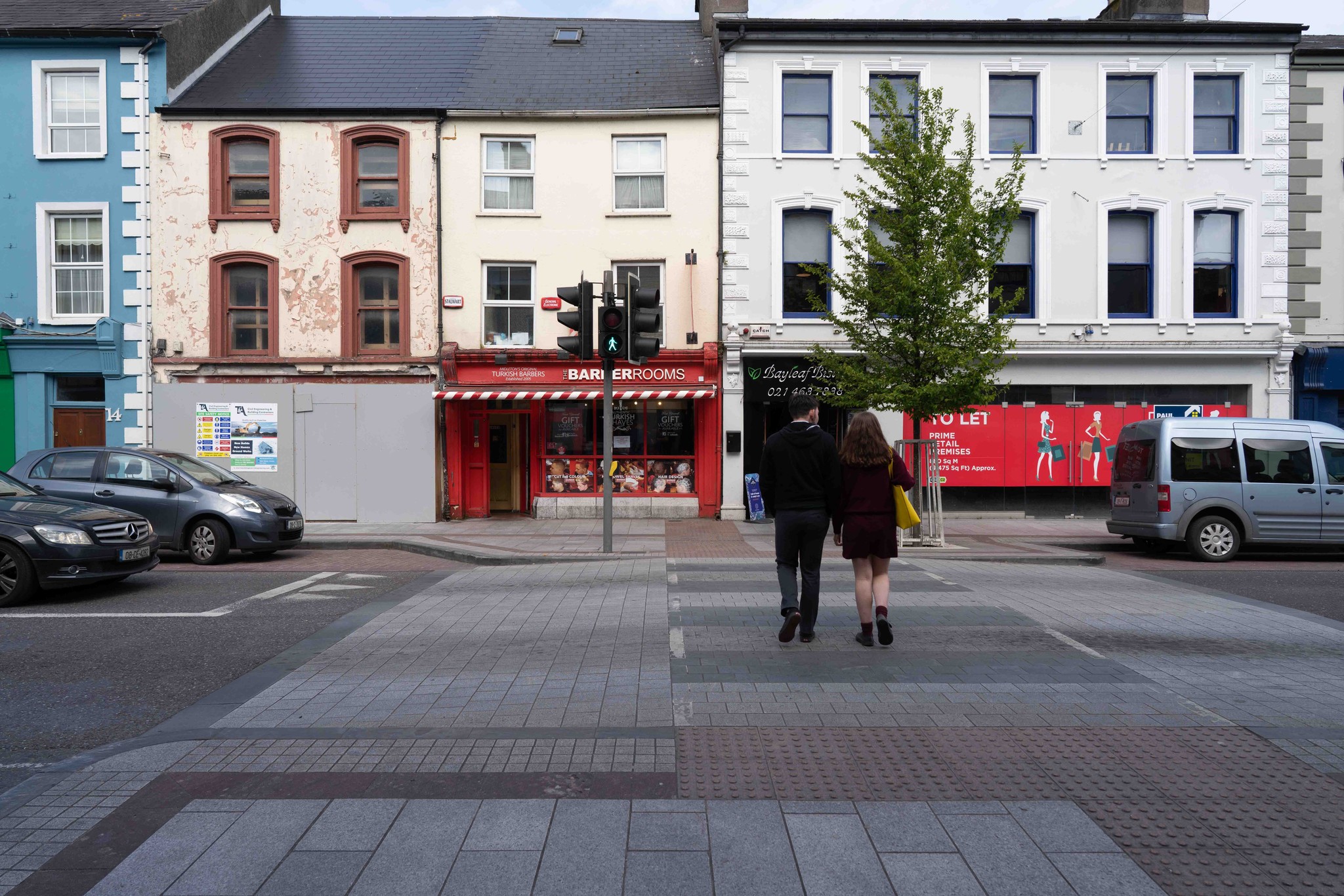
[888,446,921,530]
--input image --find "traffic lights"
[627,283,661,361]
[556,282,593,360]
[598,305,627,357]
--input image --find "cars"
[0,470,162,606]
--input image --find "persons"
[839,412,915,647]
[760,392,842,643]
[550,459,693,493]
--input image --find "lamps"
[795,267,811,277]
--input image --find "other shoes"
[800,631,816,643]
[876,614,894,645]
[778,609,802,643]
[856,633,874,646]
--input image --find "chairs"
[1254,459,1274,483]
[106,458,120,478]
[1273,459,1305,483]
[124,460,142,480]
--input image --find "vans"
[1105,417,1344,562]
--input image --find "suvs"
[5,445,304,565]
